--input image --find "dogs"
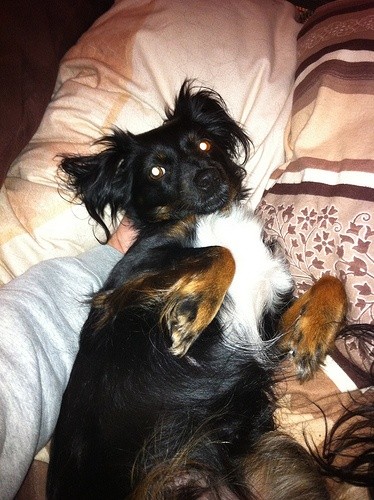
[45,79,348,500]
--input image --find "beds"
[0,0,374,500]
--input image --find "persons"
[0,212,141,500]
